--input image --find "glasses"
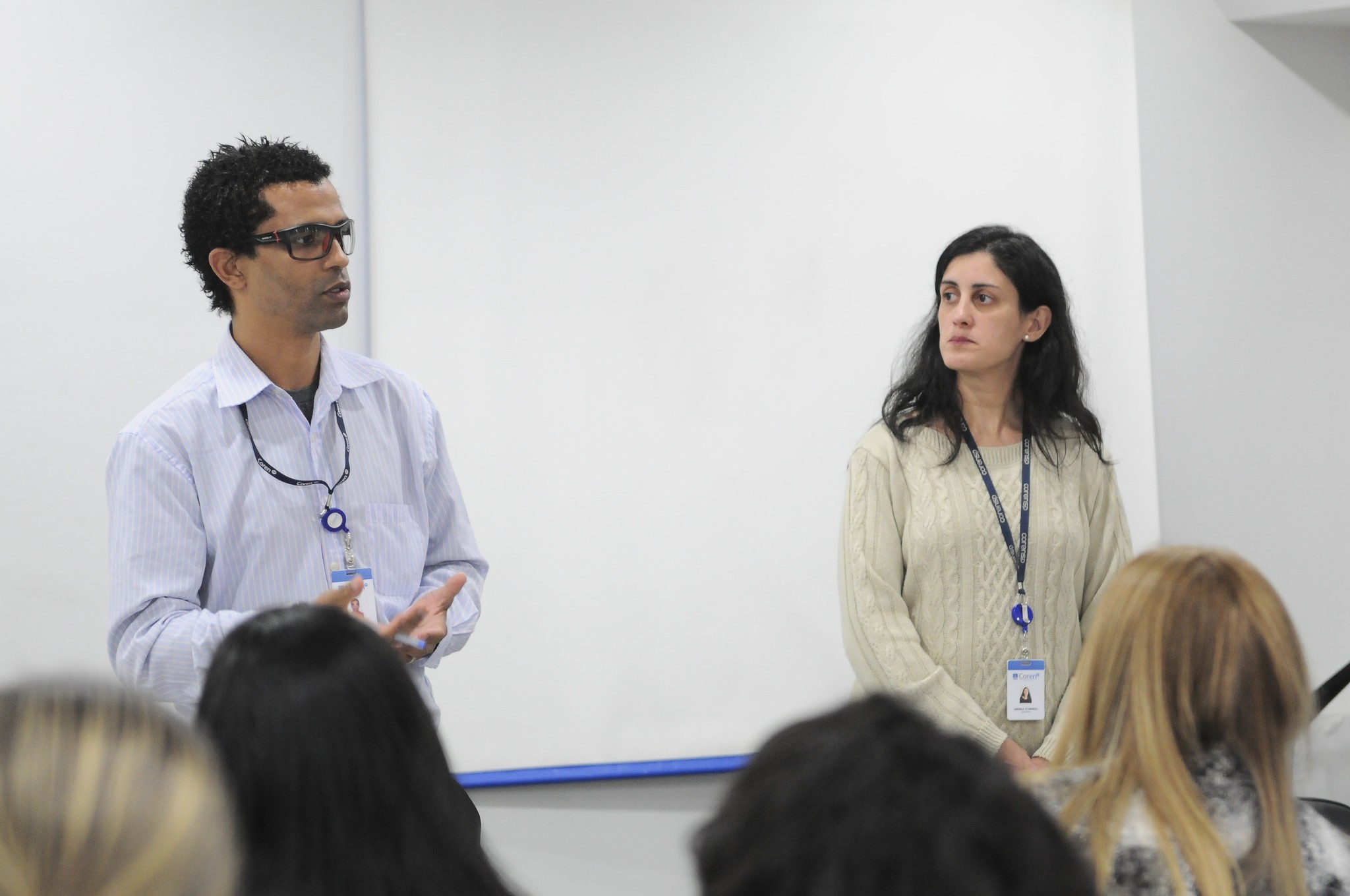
[209,219,356,261]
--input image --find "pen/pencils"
[368,624,425,649]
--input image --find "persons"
[189,607,510,896]
[105,137,495,743]
[693,698,1098,896]
[1031,544,1350,896]
[840,226,1134,771]
[1,674,249,896]
